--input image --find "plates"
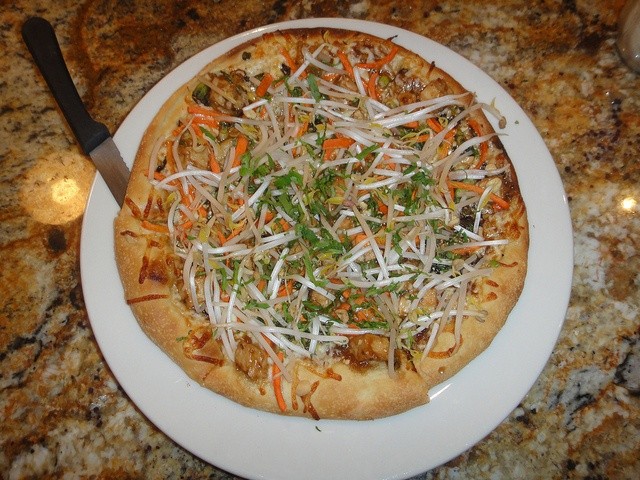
[80,17,574,476]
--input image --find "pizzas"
[113,29,529,420]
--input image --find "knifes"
[19,16,133,210]
